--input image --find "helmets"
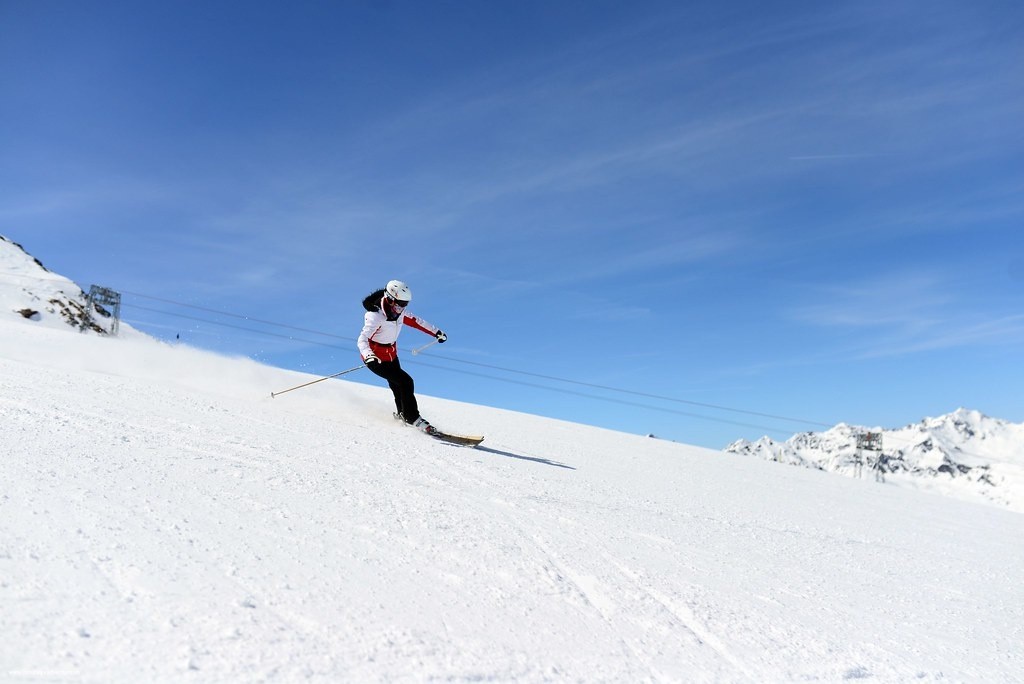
[384,279,411,315]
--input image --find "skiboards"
[392,412,485,448]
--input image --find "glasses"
[394,299,409,307]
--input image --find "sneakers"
[411,415,437,434]
[397,414,404,419]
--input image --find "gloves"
[434,329,447,343]
[365,355,382,368]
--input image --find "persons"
[359,278,449,432]
